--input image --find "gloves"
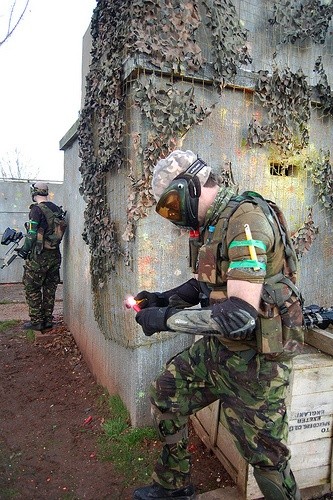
[135,278,203,313]
[135,296,176,336]
[18,246,28,259]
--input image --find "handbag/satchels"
[255,276,305,362]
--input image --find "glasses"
[29,182,48,196]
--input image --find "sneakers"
[133,480,195,500]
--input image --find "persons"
[17,181,67,330]
[135,149,303,500]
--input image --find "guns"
[302,304,333,327]
[1,226,27,269]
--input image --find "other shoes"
[41,322,52,332]
[23,322,42,331]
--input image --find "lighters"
[135,298,147,305]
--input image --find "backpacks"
[36,202,69,249]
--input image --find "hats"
[150,149,213,207]
